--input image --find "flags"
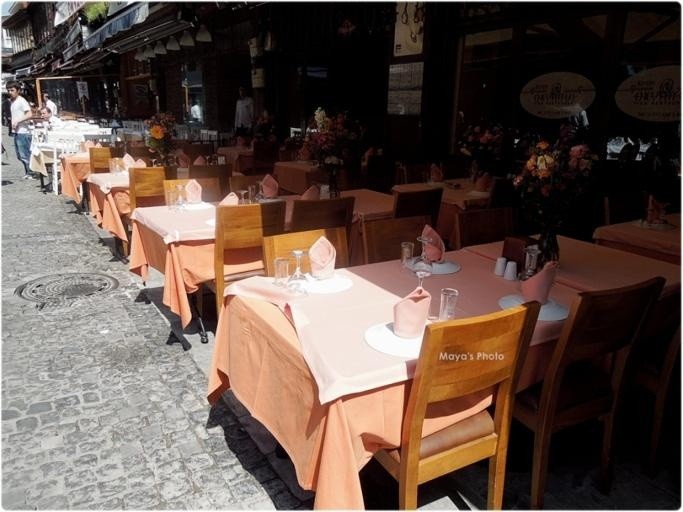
[76,80,89,100]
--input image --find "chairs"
[30,114,679,510]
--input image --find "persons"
[40,107,64,131]
[190,99,202,122]
[233,84,255,131]
[6,80,38,179]
[42,93,57,117]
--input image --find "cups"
[399,241,414,269]
[438,287,458,320]
[493,257,516,283]
[167,191,176,210]
[274,258,289,288]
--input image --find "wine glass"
[288,249,309,293]
[640,204,670,231]
[412,236,434,277]
[146,158,158,167]
[59,133,80,157]
[173,182,188,212]
[204,155,210,166]
[237,180,268,203]
[518,248,541,282]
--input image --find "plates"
[407,255,461,275]
[497,294,570,321]
[363,318,422,359]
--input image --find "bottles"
[111,129,115,143]
[178,128,188,140]
